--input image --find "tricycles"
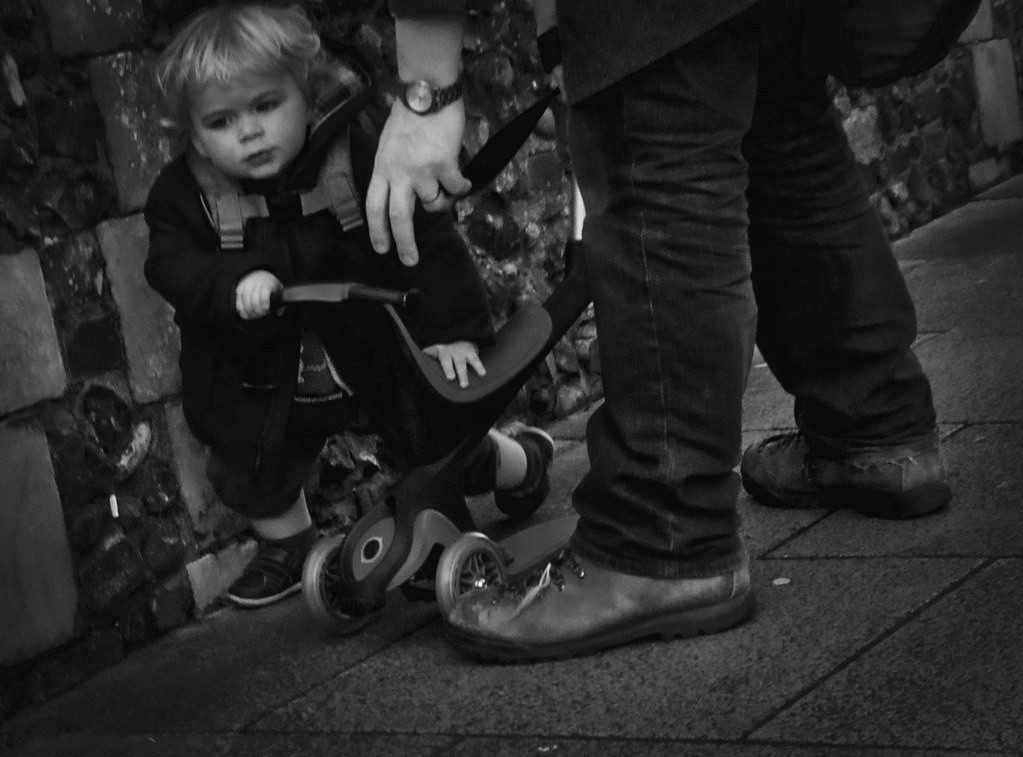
[244,36,597,649]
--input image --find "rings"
[421,186,442,204]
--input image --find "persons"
[365,0,982,663]
[143,4,554,609]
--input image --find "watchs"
[394,72,470,114]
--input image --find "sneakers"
[493,428,556,518]
[441,541,750,658]
[227,549,305,607]
[742,427,952,521]
